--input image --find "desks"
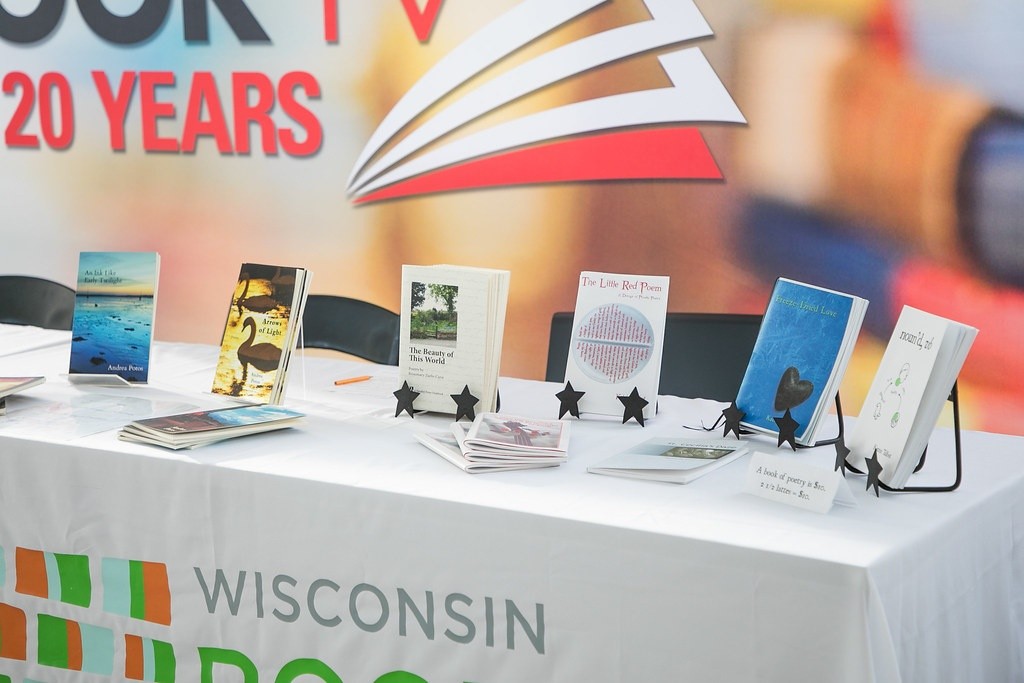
[0,322,1024,682]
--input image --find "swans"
[238,264,295,362]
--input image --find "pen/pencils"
[334,375,373,385]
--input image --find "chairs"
[297,295,401,366]
[546,313,764,403]
[0,276,77,330]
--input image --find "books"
[68,252,161,382]
[735,278,979,489]
[586,436,750,484]
[209,261,312,407]
[0,378,307,452]
[400,262,670,473]
